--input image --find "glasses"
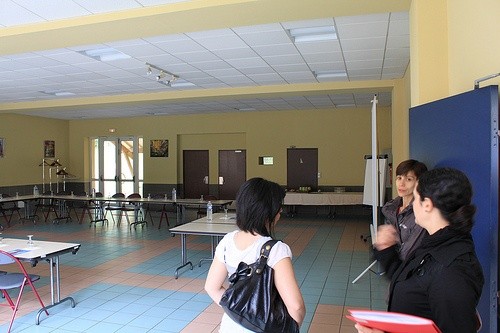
[279,207,283,213]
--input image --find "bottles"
[172,188,176,201]
[92,188,96,198]
[33,185,39,196]
[207,201,213,221]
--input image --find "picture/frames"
[44,140,55,157]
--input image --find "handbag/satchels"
[219,240,299,333]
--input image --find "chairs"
[196,194,219,218]
[0,250,49,333]
[0,194,24,227]
[32,191,182,230]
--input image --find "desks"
[37,193,92,225]
[0,236,81,325]
[281,191,363,219]
[169,222,242,280]
[194,213,241,248]
[0,195,37,228]
[89,196,177,231]
[173,198,234,216]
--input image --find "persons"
[354,168,484,333]
[204,177,306,333]
[381,159,426,262]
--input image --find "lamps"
[147,66,176,84]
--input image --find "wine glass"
[27,234,35,248]
[224,208,228,218]
[16,192,19,198]
